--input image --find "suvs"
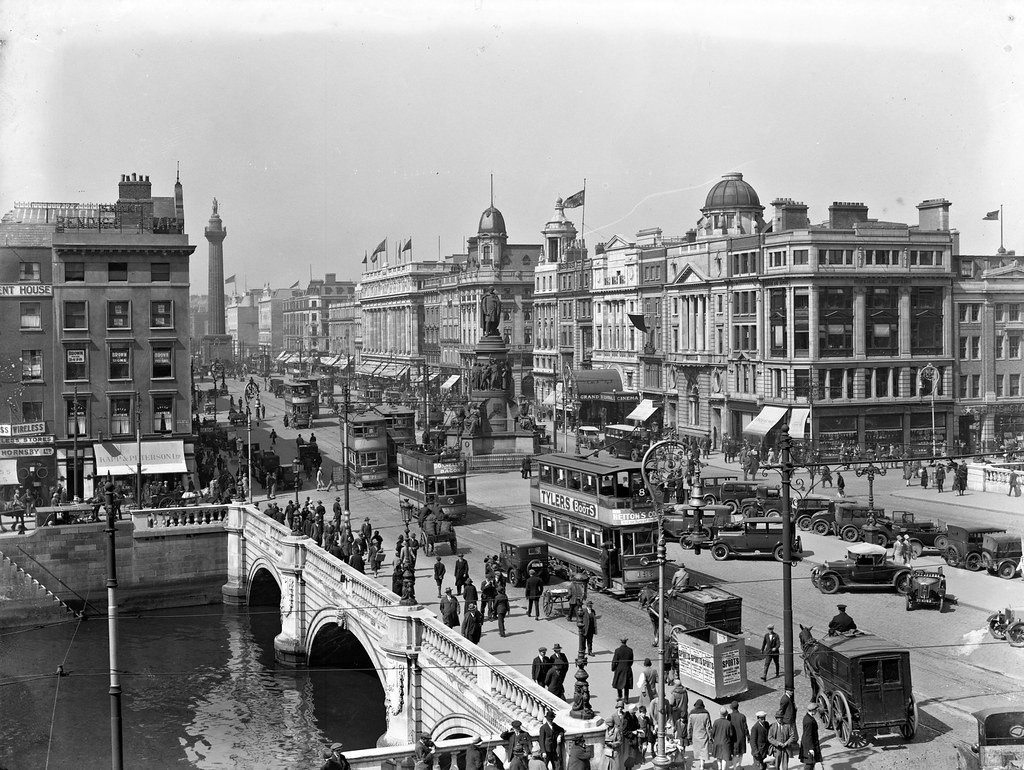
[497,539,550,587]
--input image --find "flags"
[562,190,585,208]
[289,281,299,288]
[397,243,401,259]
[982,210,999,220]
[225,275,236,283]
[362,256,367,263]
[402,240,411,251]
[372,255,377,263]
[371,240,386,257]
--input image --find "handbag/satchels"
[605,747,614,758]
[375,552,386,561]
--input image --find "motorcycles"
[986,608,1024,647]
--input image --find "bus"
[396,444,467,526]
[284,377,416,490]
[529,453,664,595]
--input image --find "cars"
[662,505,731,549]
[218,426,303,491]
[536,425,653,462]
[699,476,948,557]
[903,567,947,613]
[709,517,795,562]
[941,522,1023,579]
[810,543,913,595]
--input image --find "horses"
[798,624,820,703]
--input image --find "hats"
[572,734,585,743]
[531,746,542,756]
[473,735,483,745]
[587,601,593,606]
[838,605,847,611]
[896,534,909,540]
[511,720,522,727]
[808,702,820,711]
[678,563,686,570]
[420,732,432,739]
[270,496,506,612]
[528,569,536,575]
[513,746,525,754]
[538,624,798,727]
[332,743,343,749]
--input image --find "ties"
[542,658,544,663]
[558,654,560,659]
[517,732,519,737]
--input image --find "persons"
[11,400,1019,770]
[452,286,537,436]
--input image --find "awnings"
[743,406,788,435]
[275,350,460,388]
[542,392,573,411]
[626,399,664,421]
[92,439,188,476]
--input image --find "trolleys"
[543,589,570,617]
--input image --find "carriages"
[637,584,743,651]
[149,487,210,516]
[799,624,918,747]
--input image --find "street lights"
[641,440,707,770]
[399,498,417,606]
[569,568,595,720]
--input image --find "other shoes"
[535,618,539,621]
[499,632,506,637]
[761,677,766,682]
[526,611,531,616]
